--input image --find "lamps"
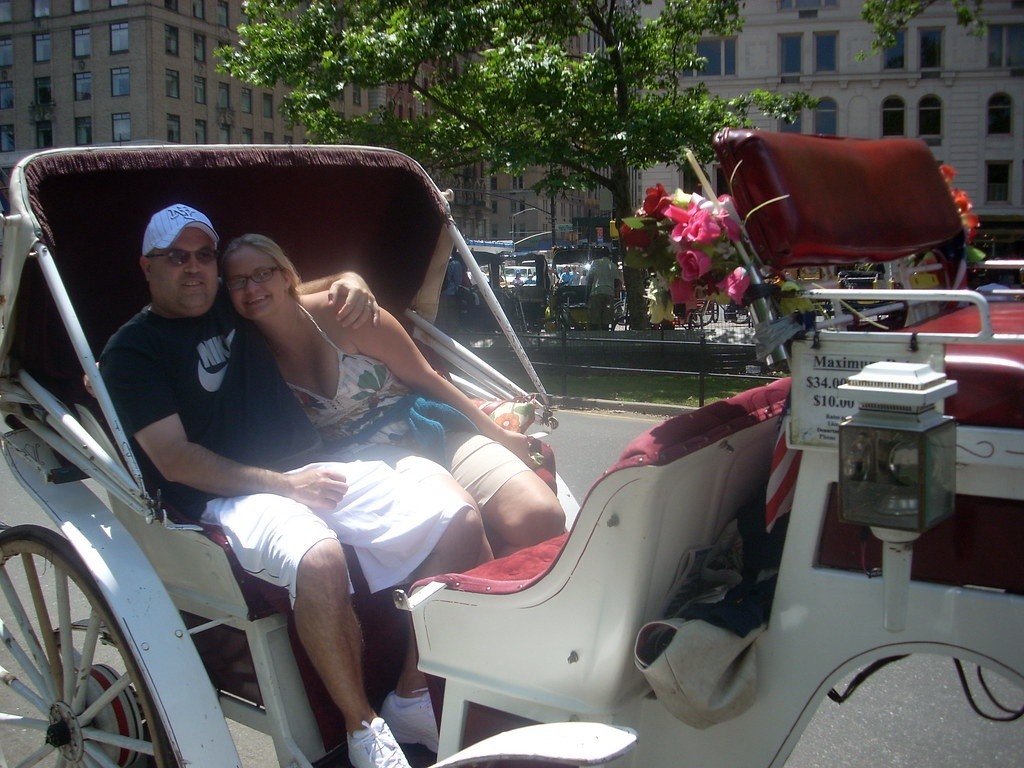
[838,361,955,634]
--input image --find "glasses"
[143,247,220,266]
[224,265,283,289]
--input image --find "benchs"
[714,128,1024,499]
[553,285,587,309]
[520,286,544,304]
[393,377,790,716]
[75,337,557,621]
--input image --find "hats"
[142,203,219,256]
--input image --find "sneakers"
[381,690,442,754]
[347,717,412,768]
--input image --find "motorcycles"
[435,237,941,334]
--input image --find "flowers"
[614,183,813,331]
[908,165,985,265]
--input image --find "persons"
[863,262,885,280]
[99,204,440,768]
[524,274,536,284]
[671,280,692,317]
[647,289,675,330]
[548,266,587,286]
[84,233,567,570]
[585,247,621,331]
[513,272,524,284]
